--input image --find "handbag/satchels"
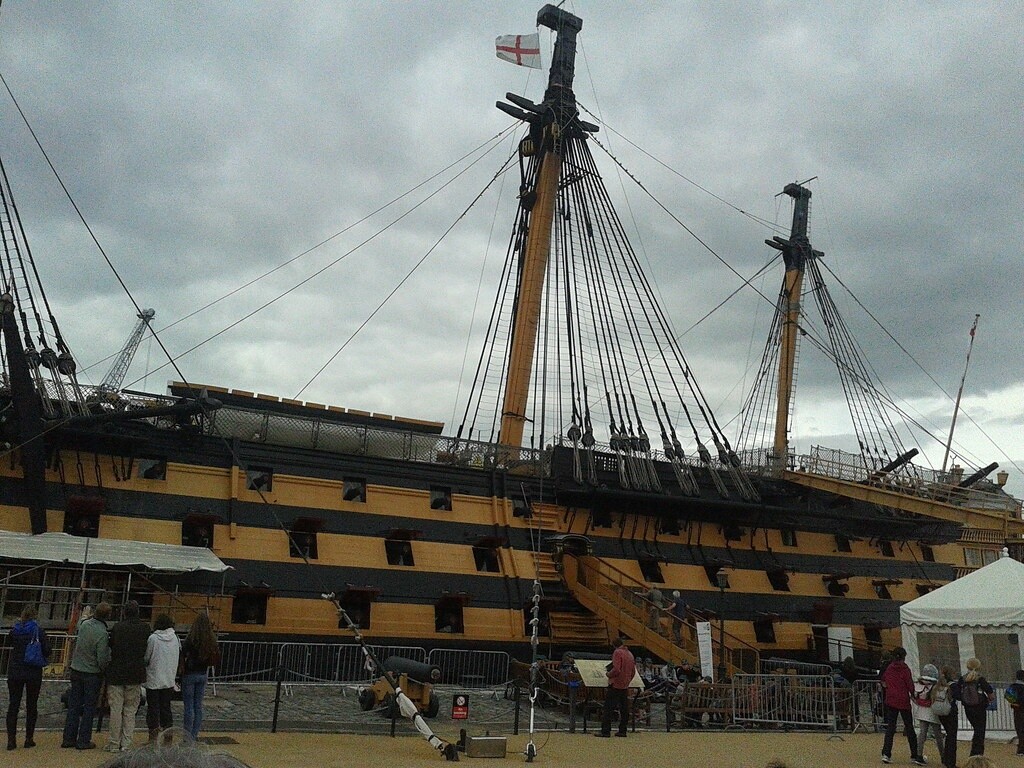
[24,628,47,676]
[606,661,613,672]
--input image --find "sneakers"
[882,754,893,764]
[912,758,916,762]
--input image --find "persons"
[594,638,636,736]
[61,602,252,768]
[4,605,53,750]
[635,583,1024,768]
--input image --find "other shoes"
[141,742,172,749]
[76,741,95,749]
[179,741,197,747]
[595,732,610,737]
[103,744,135,752]
[7,739,17,750]
[1016,750,1024,756]
[943,764,959,768]
[61,739,77,748]
[615,732,626,737]
[915,755,926,765]
[24,739,36,748]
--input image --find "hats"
[636,657,642,662]
[667,662,675,668]
[681,659,688,665]
[645,658,652,663]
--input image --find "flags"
[496,34,543,70]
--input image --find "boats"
[1,0,1023,686]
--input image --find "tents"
[900,547,1024,744]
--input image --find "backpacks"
[1004,685,1017,704]
[961,674,980,706]
[912,680,937,707]
[931,682,955,715]
